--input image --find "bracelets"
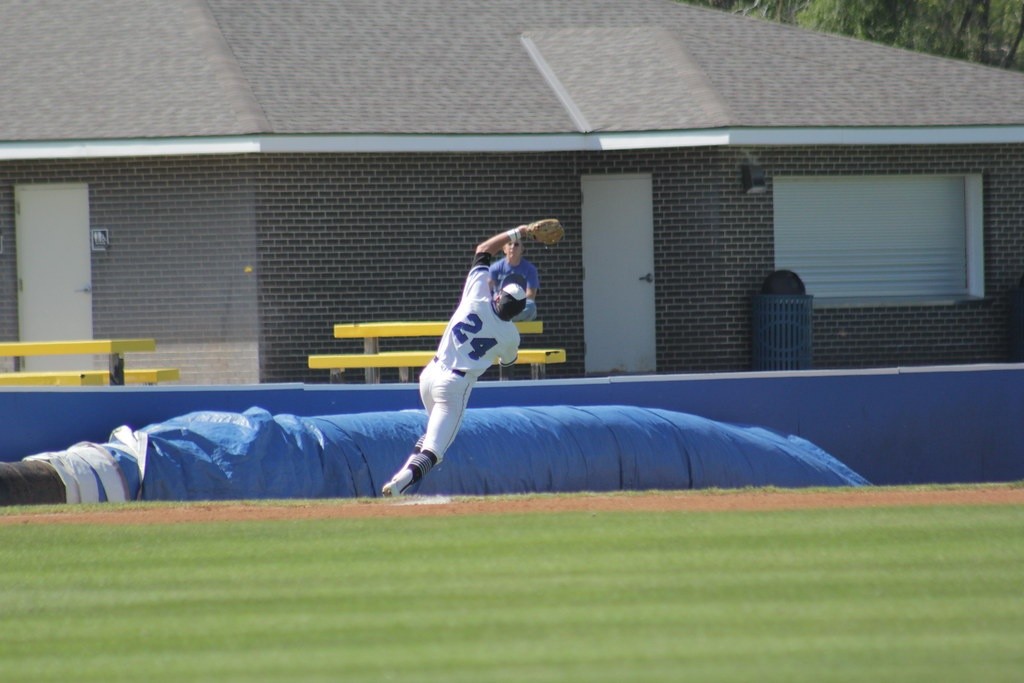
[507,228,521,241]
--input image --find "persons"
[488,238,538,321]
[381,219,564,498]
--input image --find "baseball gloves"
[527,219,564,244]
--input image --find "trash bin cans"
[747,271,814,371]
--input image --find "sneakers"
[383,482,400,498]
[392,475,398,482]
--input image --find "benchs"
[309,323,567,381]
[0,338,181,386]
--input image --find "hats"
[499,273,526,317]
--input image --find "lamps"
[741,153,768,194]
